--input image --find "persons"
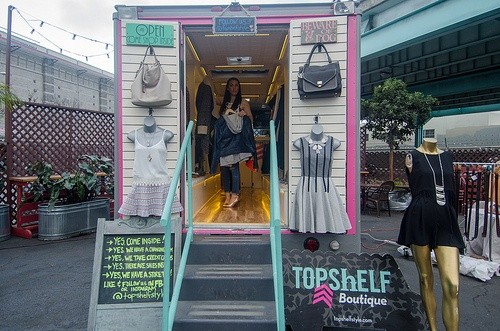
[398,138,465,331]
[117,116,184,217]
[215,77,253,208]
[195,76,214,173]
[288,125,352,234]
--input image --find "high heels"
[223,194,241,207]
[224,195,231,205]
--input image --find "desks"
[360,184,408,215]
[8,171,114,238]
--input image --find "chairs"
[363,180,396,216]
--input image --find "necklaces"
[421,145,446,206]
[145,134,153,146]
[309,136,328,154]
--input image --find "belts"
[454,164,500,240]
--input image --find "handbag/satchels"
[296,43,342,100]
[130,46,172,107]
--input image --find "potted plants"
[27,155,114,241]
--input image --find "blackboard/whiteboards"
[212,15,257,34]
[88,217,183,331]
